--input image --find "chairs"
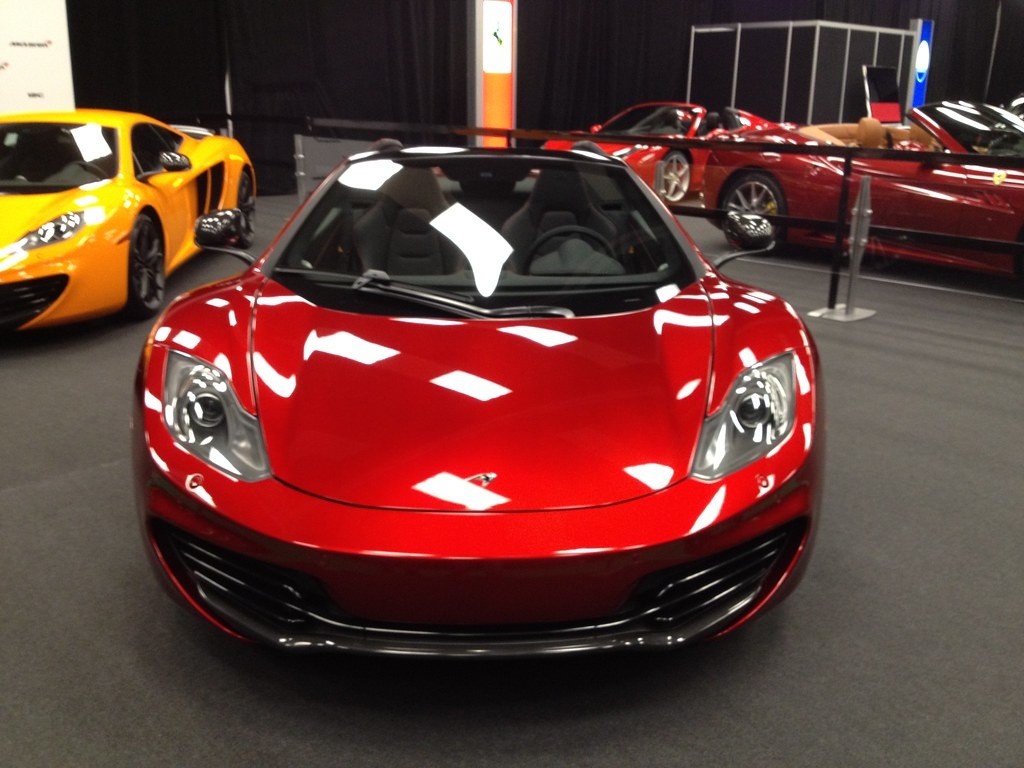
[503,164,619,277]
[856,115,883,150]
[910,123,934,150]
[351,165,465,273]
[701,110,724,134]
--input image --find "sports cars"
[0,110,258,337]
[536,100,824,206]
[132,140,826,677]
[708,92,1024,297]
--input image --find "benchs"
[804,122,910,146]
